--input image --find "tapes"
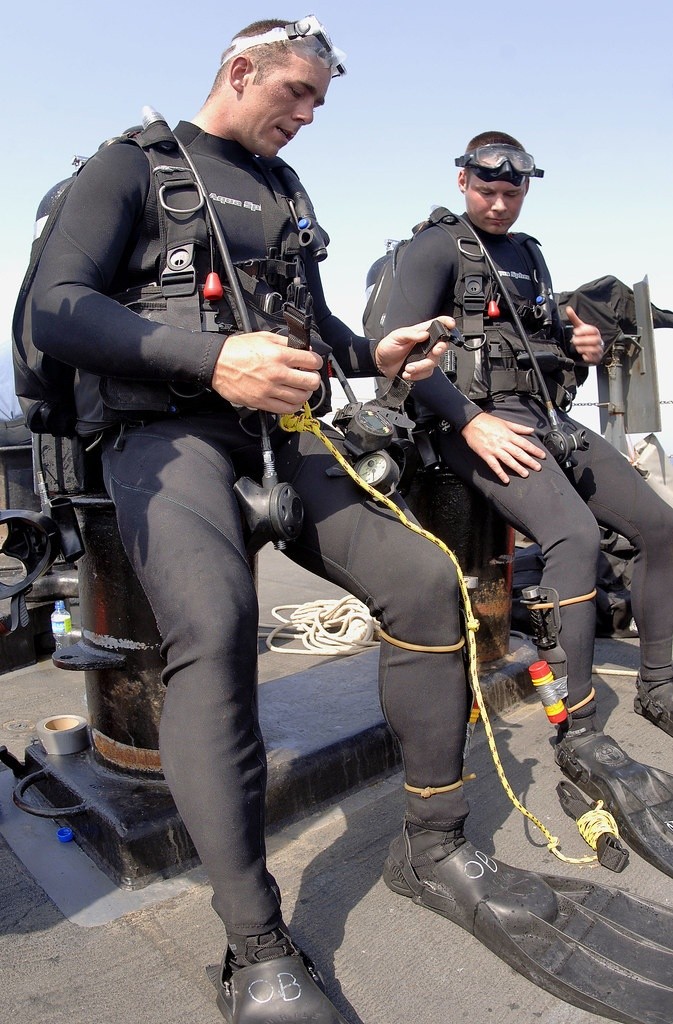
[35,715,91,755]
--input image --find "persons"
[380,131,673,807]
[32,18,557,1024]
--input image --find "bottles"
[51,600,72,651]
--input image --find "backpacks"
[11,125,311,440]
[361,206,577,406]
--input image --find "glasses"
[454,142,544,187]
[224,14,349,79]
